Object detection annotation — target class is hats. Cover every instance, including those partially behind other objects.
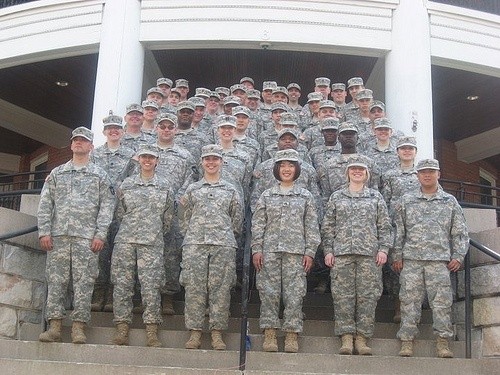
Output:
[72,77,440,171]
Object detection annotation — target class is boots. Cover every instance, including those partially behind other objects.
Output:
[103,288,114,313]
[132,303,144,314]
[355,336,373,354]
[262,327,278,351]
[90,289,104,312]
[161,293,176,315]
[184,330,203,349]
[394,299,401,322]
[38,319,62,344]
[340,334,354,355]
[70,320,86,344]
[146,323,163,348]
[211,328,226,350]
[107,323,129,345]
[435,337,453,358]
[284,332,299,353]
[399,341,412,357]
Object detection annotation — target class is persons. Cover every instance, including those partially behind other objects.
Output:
[86,77,450,324]
[249,149,321,354]
[320,163,392,355]
[39,125,117,344]
[109,144,173,350]
[391,160,472,358]
[177,140,248,350]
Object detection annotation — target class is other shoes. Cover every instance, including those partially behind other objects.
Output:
[314,280,327,293]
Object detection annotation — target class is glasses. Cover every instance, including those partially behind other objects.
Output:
[158,124,175,130]
